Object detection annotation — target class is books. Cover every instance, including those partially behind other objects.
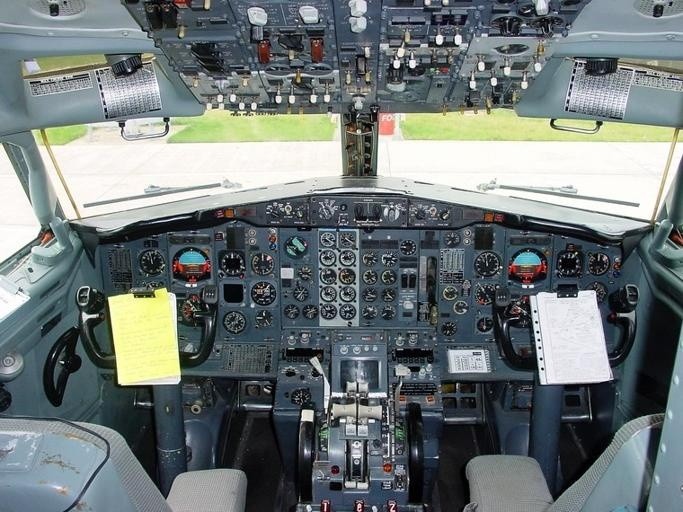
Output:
[527,289,615,387]
[105,285,182,389]
